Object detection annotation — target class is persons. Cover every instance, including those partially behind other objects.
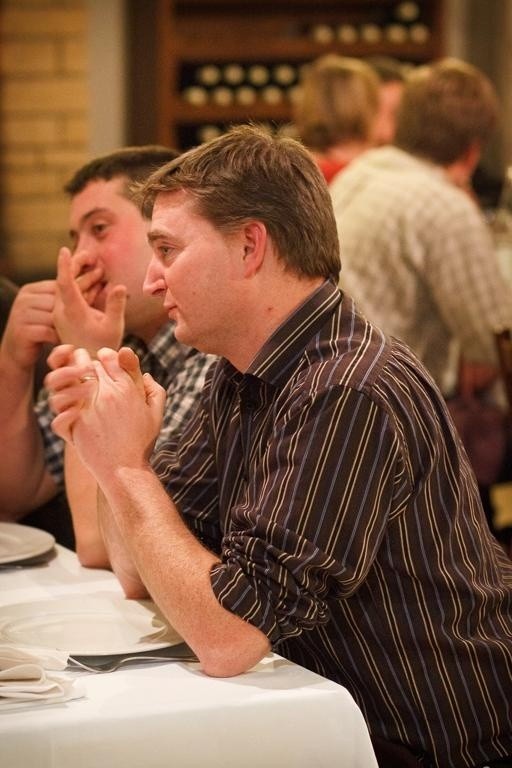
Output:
[48,127,511,766]
[2,146,223,569]
[292,56,512,485]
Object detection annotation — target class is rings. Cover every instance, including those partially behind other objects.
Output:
[79,373,96,384]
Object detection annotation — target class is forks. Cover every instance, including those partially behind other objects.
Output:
[68,655,202,673]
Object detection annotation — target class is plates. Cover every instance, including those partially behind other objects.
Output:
[0,594,186,656]
[0,519,54,564]
